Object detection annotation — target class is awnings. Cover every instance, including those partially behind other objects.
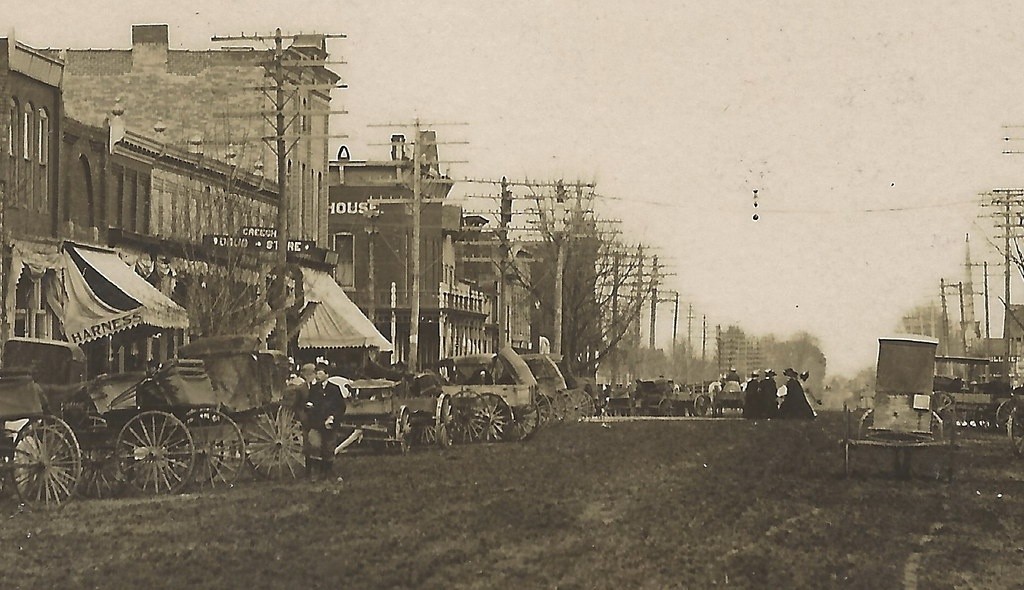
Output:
[58,242,190,349]
[300,268,394,354]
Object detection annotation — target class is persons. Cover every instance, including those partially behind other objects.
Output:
[726,367,741,383]
[776,367,817,419]
[594,373,681,417]
[308,365,345,487]
[757,369,779,418]
[293,363,317,482]
[741,369,761,418]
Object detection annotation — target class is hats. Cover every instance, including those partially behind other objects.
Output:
[764,368,777,377]
[783,368,798,378]
[731,367,736,372]
[751,369,760,377]
[315,362,328,374]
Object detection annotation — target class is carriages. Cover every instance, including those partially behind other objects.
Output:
[0,333,1024,514]
